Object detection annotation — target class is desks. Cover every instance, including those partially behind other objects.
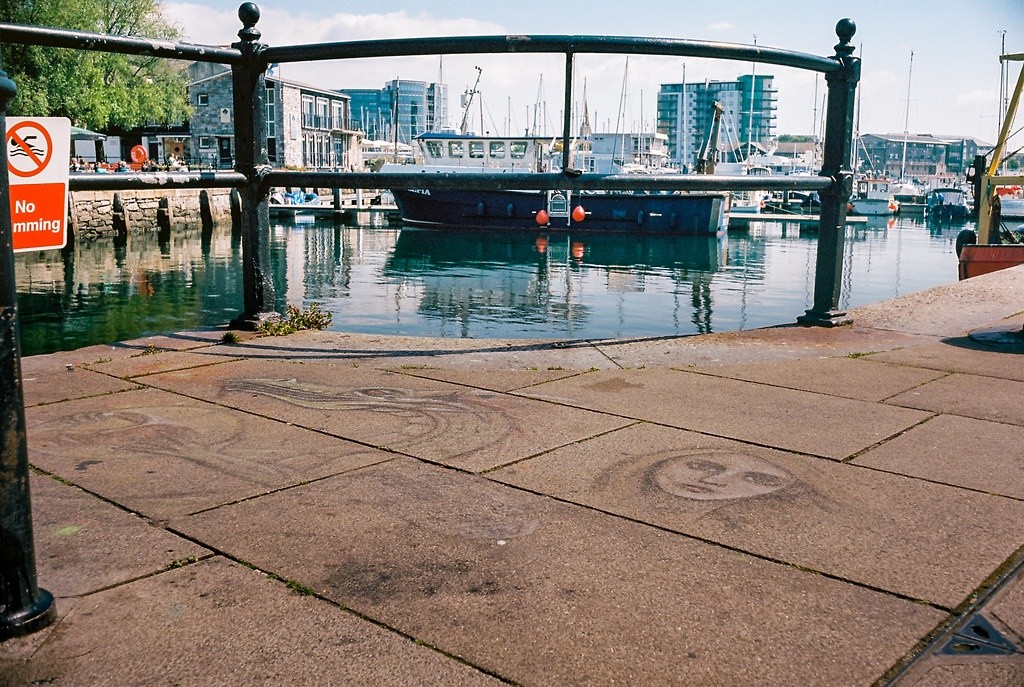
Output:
[157,165,213,172]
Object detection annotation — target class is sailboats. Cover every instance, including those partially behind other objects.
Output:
[268,29,1023,232]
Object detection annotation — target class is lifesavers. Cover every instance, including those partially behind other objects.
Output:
[130,144,147,164]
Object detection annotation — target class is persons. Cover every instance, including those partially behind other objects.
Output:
[168,153,181,173]
[115,161,129,172]
[69,157,80,171]
[95,162,109,174]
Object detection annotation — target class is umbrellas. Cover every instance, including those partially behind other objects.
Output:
[70,126,107,141]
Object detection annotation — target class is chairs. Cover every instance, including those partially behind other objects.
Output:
[74,156,218,173]
